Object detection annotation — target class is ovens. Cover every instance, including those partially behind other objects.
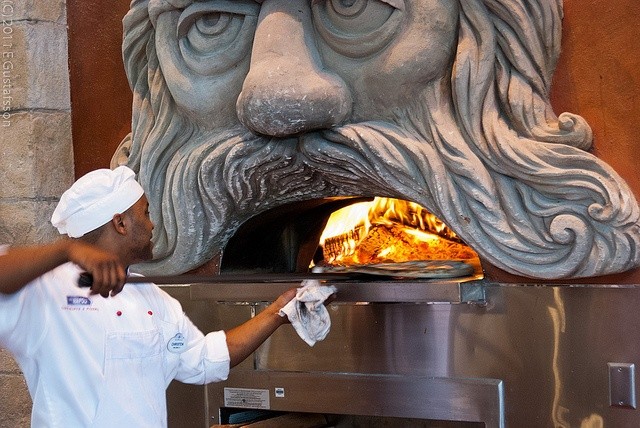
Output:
[118,273,638,428]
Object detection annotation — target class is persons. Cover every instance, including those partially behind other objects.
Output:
[0,165,337,428]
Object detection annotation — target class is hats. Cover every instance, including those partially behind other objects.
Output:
[51,166,145,239]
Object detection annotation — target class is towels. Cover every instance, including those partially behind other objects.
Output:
[279,272,339,352]
